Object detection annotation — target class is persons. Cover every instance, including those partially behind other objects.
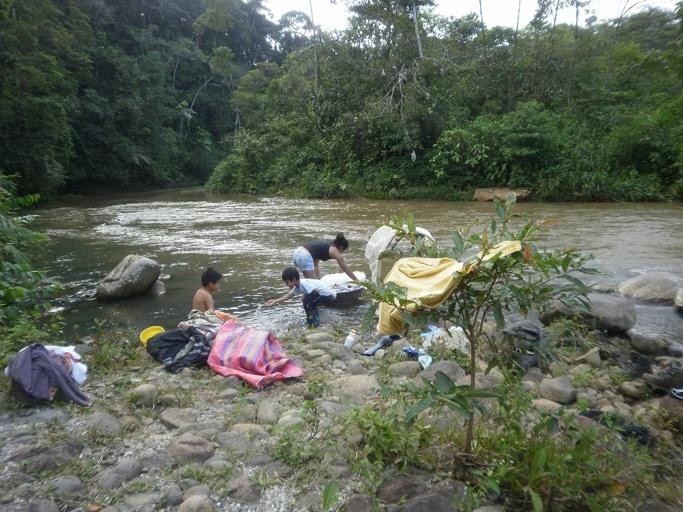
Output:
[191,267,224,314]
[264,266,336,330]
[289,232,365,285]
